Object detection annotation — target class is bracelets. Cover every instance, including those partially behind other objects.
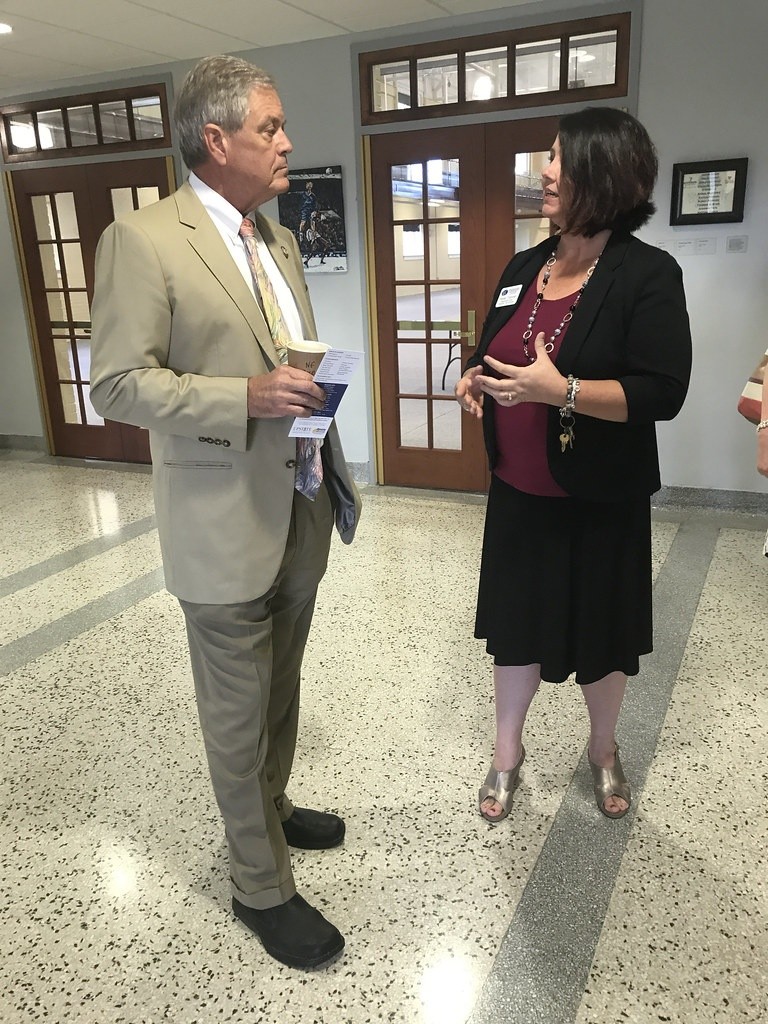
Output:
[756,420,768,432]
[558,372,580,417]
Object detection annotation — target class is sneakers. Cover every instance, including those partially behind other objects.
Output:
[231,892,346,971]
[283,806,346,849]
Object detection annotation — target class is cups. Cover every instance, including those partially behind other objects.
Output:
[285,341,335,376]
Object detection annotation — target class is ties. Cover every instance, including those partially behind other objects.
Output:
[240,217,323,502]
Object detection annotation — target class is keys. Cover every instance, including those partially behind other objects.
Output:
[557,416,575,453]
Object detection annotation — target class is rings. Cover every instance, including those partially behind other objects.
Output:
[507,393,512,401]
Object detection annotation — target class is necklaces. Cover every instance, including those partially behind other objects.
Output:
[522,246,604,363]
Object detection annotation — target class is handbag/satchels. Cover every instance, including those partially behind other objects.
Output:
[738,349,768,426]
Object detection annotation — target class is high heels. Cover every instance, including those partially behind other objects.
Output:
[479,743,525,822]
[588,741,631,818]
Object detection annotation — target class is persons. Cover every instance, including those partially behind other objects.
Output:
[87,52,362,969]
[454,107,694,823]
[298,182,330,269]
[756,363,768,480]
